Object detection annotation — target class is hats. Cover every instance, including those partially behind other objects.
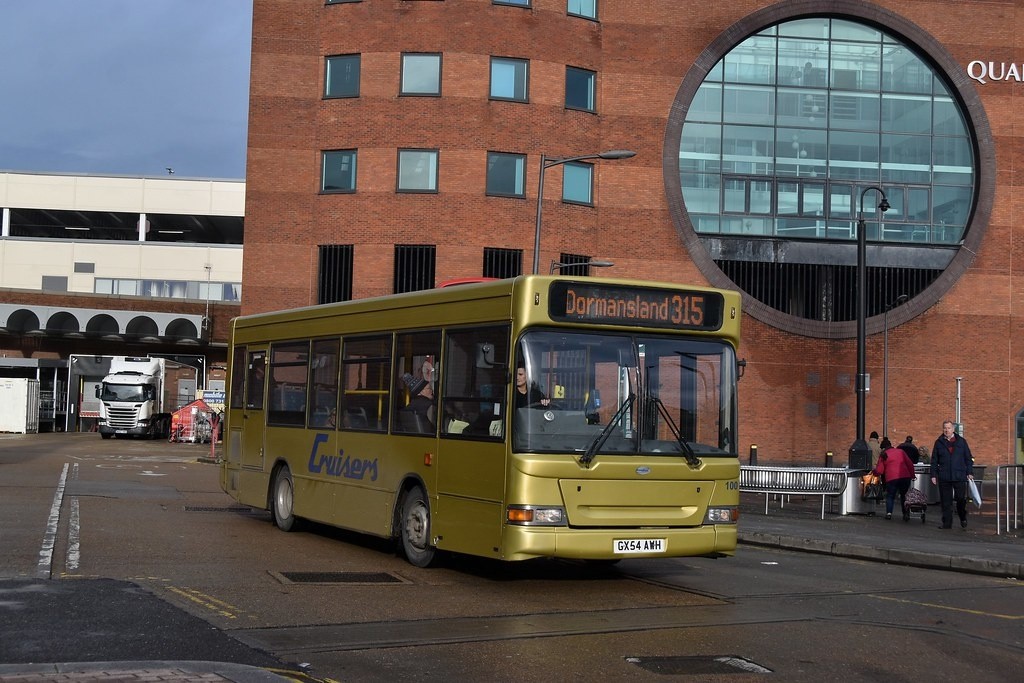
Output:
[870,431,878,439]
[402,372,429,395]
[880,437,891,448]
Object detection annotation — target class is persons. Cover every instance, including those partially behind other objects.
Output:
[868,431,930,469]
[403,373,438,433]
[875,437,917,521]
[325,408,336,428]
[240,358,276,406]
[515,368,550,410]
[930,420,974,529]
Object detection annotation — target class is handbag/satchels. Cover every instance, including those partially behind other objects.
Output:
[863,472,883,500]
[969,477,981,508]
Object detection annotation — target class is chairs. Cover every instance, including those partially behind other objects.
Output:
[234,379,471,434]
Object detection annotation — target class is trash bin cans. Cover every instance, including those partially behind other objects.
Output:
[838,469,876,516]
[910,462,940,503]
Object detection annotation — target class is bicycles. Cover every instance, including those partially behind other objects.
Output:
[168,426,184,443]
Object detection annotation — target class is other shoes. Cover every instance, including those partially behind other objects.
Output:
[939,524,951,530]
[886,512,892,519]
[960,517,967,528]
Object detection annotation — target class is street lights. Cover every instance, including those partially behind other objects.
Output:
[531,151,638,274]
[882,294,909,438]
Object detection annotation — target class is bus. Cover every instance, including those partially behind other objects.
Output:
[219,273,747,567]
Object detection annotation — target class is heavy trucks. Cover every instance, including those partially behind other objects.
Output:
[95,357,199,440]
